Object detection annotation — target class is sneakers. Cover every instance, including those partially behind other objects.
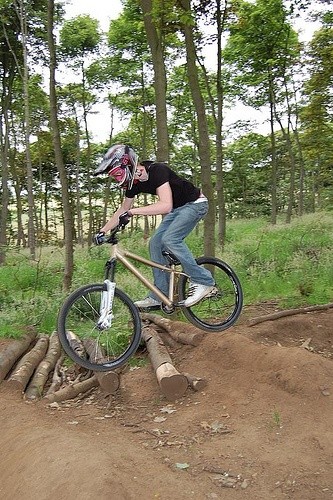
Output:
[133,297,166,310]
[185,283,214,307]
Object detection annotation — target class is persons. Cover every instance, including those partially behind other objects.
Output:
[92,144,215,307]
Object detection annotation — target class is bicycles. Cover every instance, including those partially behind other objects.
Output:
[57,211,244,372]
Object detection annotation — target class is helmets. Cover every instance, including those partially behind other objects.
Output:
[93,144,139,192]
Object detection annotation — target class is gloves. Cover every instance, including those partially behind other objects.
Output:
[94,232,106,245]
[117,212,130,226]
